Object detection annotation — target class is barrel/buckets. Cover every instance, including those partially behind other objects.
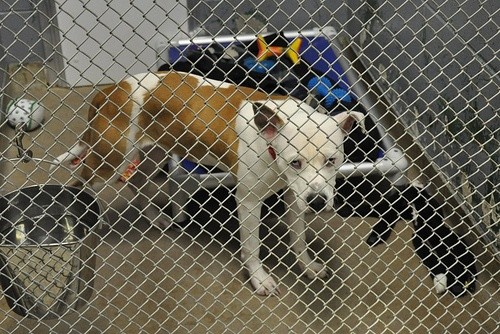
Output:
[1,184,102,320]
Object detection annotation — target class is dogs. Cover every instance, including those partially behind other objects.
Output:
[49,71,366,297]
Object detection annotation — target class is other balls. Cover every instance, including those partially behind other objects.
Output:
[6,97,44,132]
